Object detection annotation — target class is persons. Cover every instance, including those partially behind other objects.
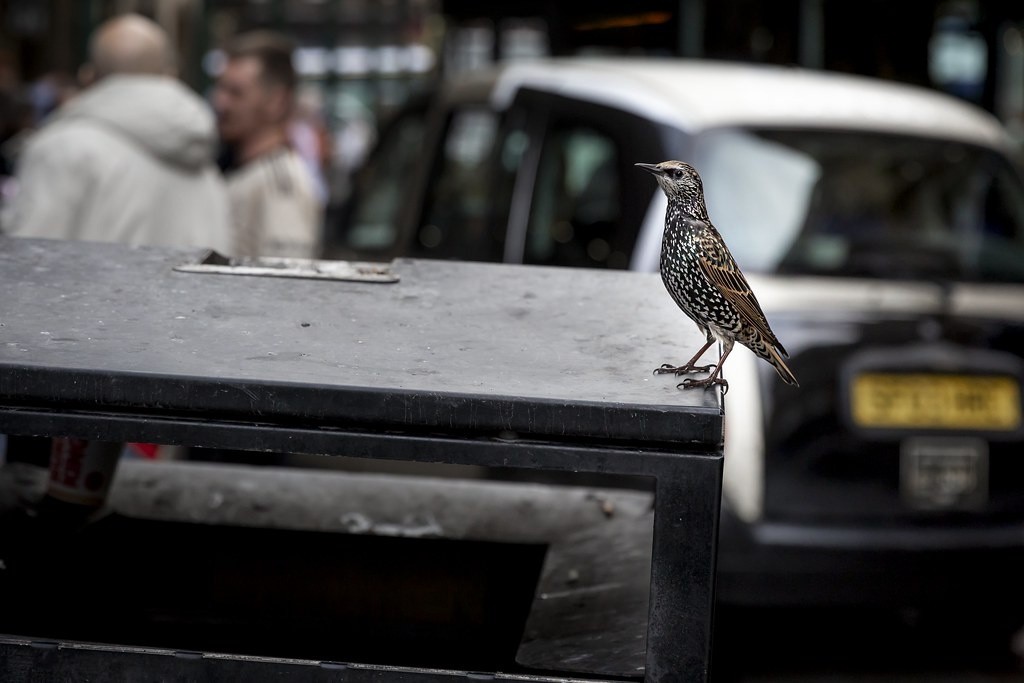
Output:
[1,11,232,253]
[213,29,329,257]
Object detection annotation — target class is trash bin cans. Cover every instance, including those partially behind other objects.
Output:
[0,237,724,683]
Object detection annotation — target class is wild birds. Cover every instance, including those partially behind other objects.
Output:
[634,159,800,394]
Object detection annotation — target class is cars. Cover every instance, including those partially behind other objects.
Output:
[316,54,1024,683]
